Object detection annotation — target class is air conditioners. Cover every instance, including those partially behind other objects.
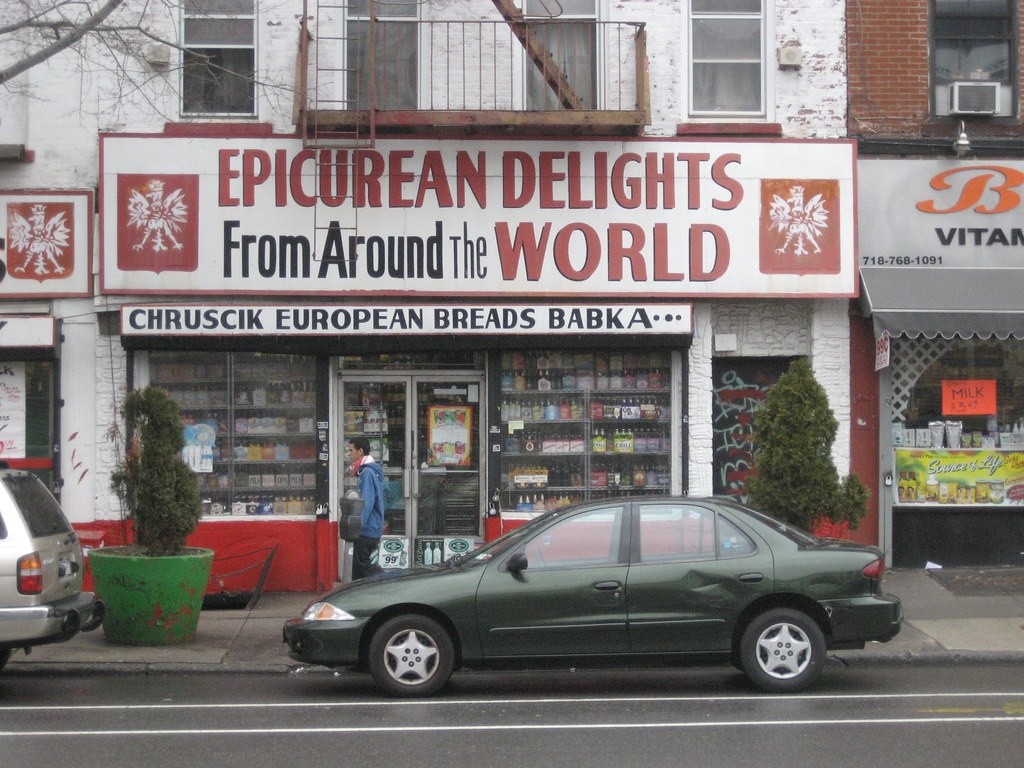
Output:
[948,80,1003,119]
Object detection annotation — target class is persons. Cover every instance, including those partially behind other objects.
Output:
[347,436,388,578]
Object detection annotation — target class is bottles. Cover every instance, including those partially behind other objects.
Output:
[150,344,677,521]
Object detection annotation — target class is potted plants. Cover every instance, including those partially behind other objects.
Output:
[85,385,214,647]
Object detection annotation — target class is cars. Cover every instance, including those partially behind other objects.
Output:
[0,461,103,672]
[279,495,904,699]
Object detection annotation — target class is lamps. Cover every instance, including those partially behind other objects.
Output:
[952,120,970,157]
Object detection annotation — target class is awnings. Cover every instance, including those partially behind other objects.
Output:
[857,265,1024,345]
